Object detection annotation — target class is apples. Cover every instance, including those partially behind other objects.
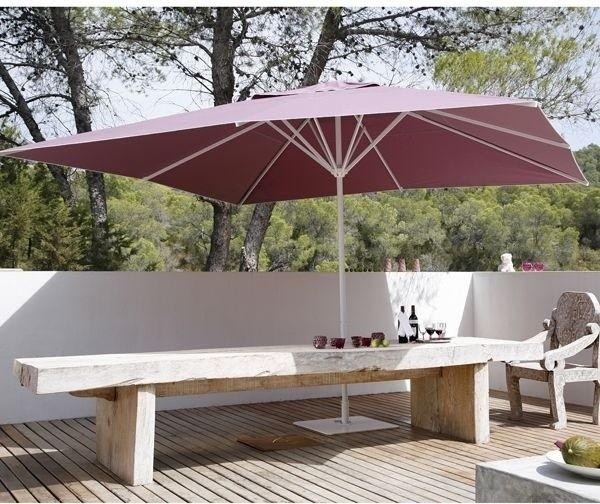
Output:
[382,339,389,347]
[371,339,379,347]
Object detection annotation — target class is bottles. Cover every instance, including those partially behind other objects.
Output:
[398,304,409,344]
[383,255,421,272]
[409,305,418,341]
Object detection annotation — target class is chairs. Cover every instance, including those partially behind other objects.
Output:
[501,289,598,432]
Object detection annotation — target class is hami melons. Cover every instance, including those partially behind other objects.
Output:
[562,435,600,468]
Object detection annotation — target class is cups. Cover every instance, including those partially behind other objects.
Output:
[522,262,545,271]
[312,332,387,350]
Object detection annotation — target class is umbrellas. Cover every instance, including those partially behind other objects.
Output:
[0,77,590,436]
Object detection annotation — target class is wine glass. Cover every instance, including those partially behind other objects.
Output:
[418,321,447,342]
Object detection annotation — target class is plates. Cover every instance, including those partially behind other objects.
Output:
[545,441,600,482]
